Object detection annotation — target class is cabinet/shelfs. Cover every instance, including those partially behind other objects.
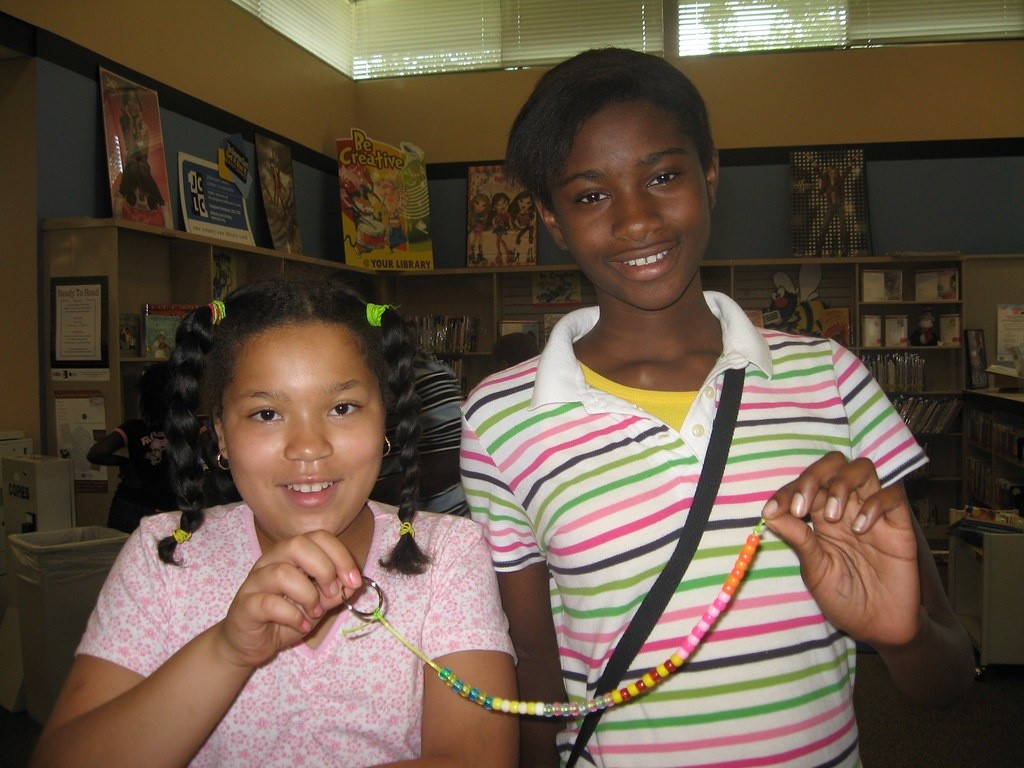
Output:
[40,211,1024,677]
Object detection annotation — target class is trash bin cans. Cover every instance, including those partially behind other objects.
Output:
[7,525,131,692]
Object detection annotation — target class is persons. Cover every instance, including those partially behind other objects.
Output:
[28,281,520,768]
[490,332,538,374]
[460,47,975,768]
[87,364,228,535]
[118,85,165,213]
[368,352,471,519]
[806,154,853,258]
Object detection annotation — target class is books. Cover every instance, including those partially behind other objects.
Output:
[968,407,1024,464]
[902,439,933,480]
[403,313,480,354]
[889,395,964,433]
[861,352,925,393]
[446,359,472,400]
[959,505,1024,534]
[968,457,1024,519]
[140,303,201,358]
[909,496,938,529]
